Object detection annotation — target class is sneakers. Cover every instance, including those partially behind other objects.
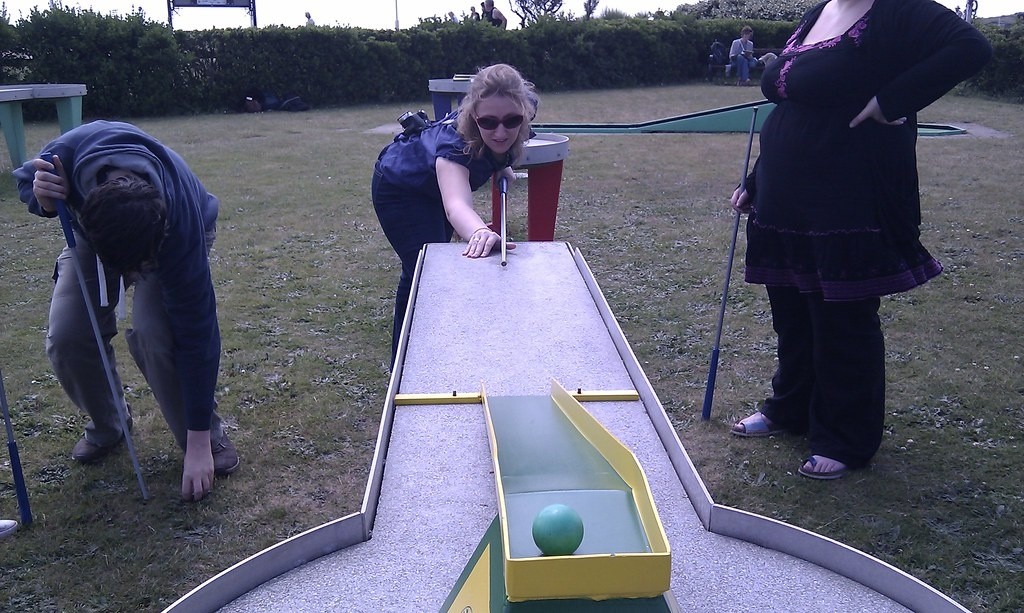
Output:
[0,520,18,538]
[211,431,239,474]
[71,402,133,463]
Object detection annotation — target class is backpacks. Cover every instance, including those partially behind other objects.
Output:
[711,42,728,64]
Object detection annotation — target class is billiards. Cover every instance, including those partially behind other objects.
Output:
[532,504,584,556]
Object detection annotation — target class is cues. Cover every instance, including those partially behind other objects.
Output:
[702,106,759,419]
[499,177,507,267]
[1,371,33,528]
[40,151,151,501]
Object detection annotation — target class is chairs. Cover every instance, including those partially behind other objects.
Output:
[707,40,765,81]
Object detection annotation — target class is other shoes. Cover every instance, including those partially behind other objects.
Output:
[744,79,751,85]
[737,81,742,86]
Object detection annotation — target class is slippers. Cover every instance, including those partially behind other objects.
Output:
[798,455,849,480]
[730,411,788,436]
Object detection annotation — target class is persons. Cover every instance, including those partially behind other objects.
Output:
[729,25,756,87]
[305,12,314,26]
[12,119,240,501]
[731,0,991,479]
[955,5,963,18]
[448,0,508,30]
[372,63,538,382]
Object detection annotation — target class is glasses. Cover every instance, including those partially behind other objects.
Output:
[475,112,523,130]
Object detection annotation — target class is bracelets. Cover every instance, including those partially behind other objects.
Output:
[471,227,492,235]
